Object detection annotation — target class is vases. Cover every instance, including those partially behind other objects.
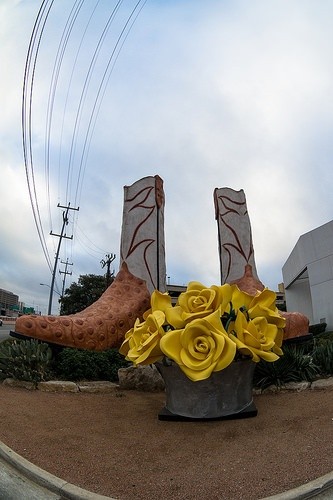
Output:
[152,361,259,422]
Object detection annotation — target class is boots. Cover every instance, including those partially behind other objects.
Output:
[214,186,313,344]
[10,174,168,352]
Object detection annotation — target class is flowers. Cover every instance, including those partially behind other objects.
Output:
[118,280,287,382]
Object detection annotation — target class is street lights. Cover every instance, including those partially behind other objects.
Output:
[39,282,64,315]
